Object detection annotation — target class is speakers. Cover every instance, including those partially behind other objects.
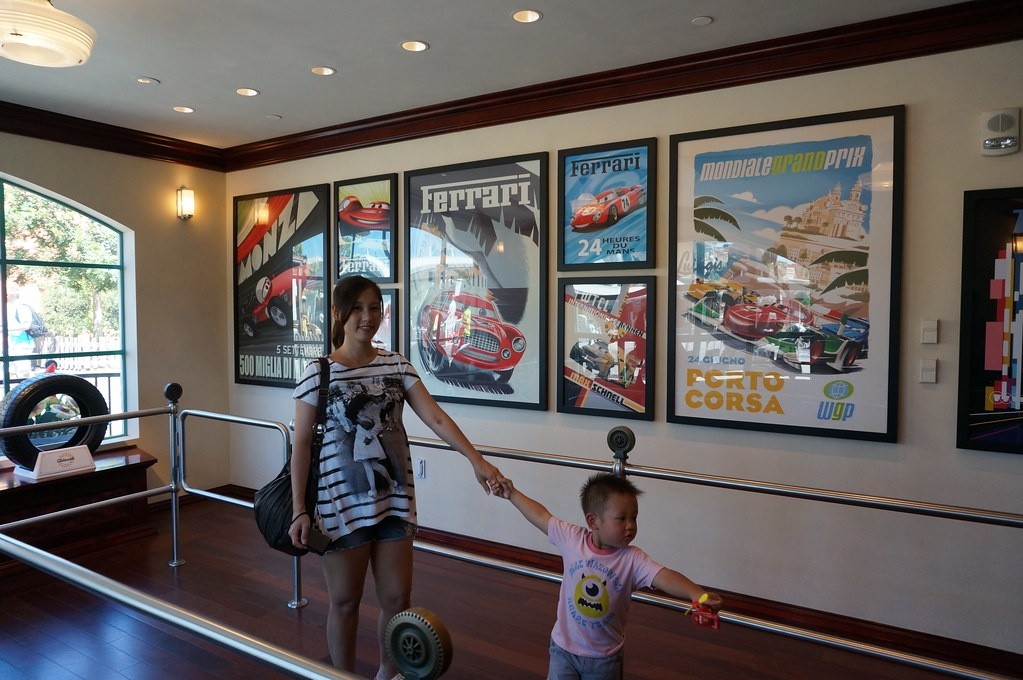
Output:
[979,108,1021,157]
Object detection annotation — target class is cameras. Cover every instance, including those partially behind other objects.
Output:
[298,529,332,556]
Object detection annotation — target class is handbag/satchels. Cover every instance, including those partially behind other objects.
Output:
[253,432,324,556]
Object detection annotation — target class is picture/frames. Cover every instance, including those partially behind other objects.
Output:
[370,288,399,353]
[403,151,549,412]
[666,103,906,445]
[233,182,331,389]
[956,186,1023,454]
[334,173,399,284]
[557,137,658,272]
[557,275,657,421]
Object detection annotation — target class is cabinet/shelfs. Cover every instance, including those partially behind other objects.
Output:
[0,444,159,578]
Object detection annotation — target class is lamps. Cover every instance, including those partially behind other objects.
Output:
[0,0,97,68]
[176,186,194,221]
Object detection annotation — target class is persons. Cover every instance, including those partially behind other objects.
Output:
[289,276,513,680]
[491,473,723,678]
